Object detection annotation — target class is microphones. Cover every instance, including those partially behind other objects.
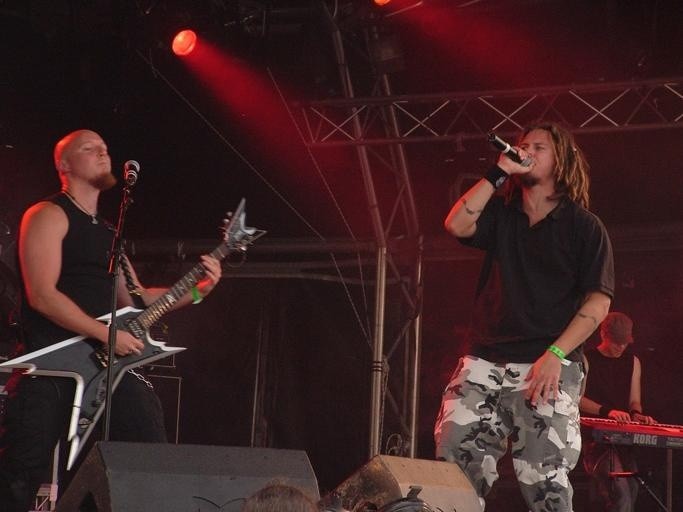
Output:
[489,133,532,167]
[124,160,140,184]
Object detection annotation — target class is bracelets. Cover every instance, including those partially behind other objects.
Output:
[548,345,566,361]
[484,163,510,189]
[631,410,641,415]
[599,407,610,418]
[191,287,203,305]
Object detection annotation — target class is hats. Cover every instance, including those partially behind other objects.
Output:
[601,312,634,344]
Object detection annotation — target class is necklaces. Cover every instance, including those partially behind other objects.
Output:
[62,189,99,224]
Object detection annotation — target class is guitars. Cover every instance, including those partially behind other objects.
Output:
[1,198,267,474]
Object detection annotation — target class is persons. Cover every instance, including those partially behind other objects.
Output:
[431,119,615,512]
[0,129,222,512]
[580,312,657,512]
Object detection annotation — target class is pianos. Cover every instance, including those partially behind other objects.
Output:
[578,416,683,451]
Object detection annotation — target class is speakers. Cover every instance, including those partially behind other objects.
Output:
[320,453,484,511]
[51,440,320,512]
